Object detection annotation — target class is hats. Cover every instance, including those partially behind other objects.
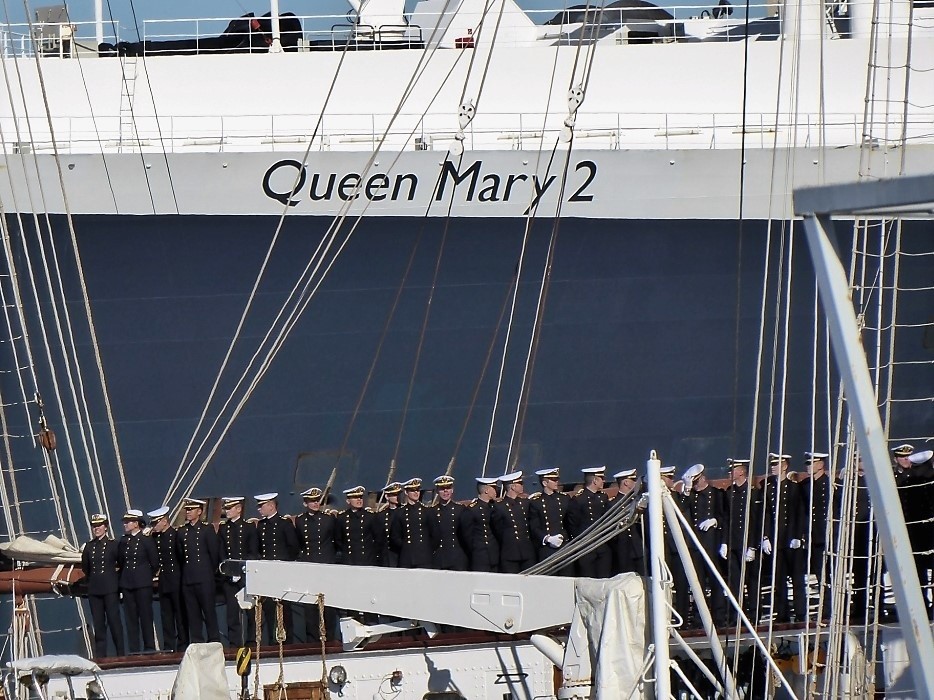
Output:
[475,466,676,487]
[300,488,323,501]
[91,514,109,526]
[343,475,455,497]
[122,510,144,521]
[254,493,278,507]
[804,444,934,468]
[147,506,170,524]
[727,458,750,473]
[769,453,792,466]
[222,497,245,510]
[183,498,207,509]
[682,464,704,485]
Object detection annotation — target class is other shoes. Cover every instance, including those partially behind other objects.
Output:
[774,617,790,623]
[794,617,805,621]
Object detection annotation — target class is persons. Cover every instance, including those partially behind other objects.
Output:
[81,445,934,659]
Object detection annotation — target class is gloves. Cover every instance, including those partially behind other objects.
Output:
[684,473,694,489]
[720,544,727,559]
[763,536,772,554]
[790,539,801,549]
[548,534,563,548]
[746,547,756,562]
[699,518,716,531]
